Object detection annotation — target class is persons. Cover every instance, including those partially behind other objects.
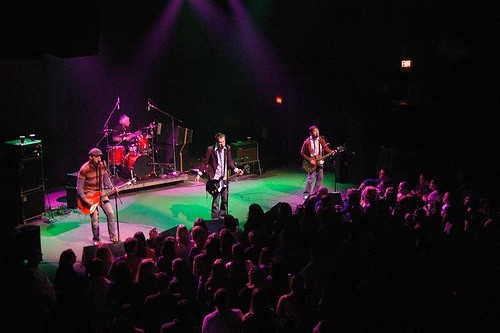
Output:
[25,167,500,333]
[77,147,120,245]
[112,115,135,146]
[300,125,335,200]
[195,133,243,220]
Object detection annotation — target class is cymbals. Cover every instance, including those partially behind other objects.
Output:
[96,129,117,134]
[142,125,160,130]
[116,132,132,138]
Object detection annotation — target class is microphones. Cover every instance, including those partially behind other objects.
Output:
[117,97,120,109]
[148,100,150,111]
[222,146,227,150]
[148,163,151,165]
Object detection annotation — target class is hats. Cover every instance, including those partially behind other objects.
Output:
[89,148,104,156]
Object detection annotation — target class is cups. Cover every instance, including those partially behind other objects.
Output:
[20,136,25,143]
[30,134,36,141]
[247,137,251,142]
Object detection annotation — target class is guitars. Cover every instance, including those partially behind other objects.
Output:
[77,177,137,215]
[302,146,344,173]
[205,164,252,198]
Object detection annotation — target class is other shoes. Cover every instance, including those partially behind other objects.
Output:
[93,239,100,246]
[111,237,120,241]
[302,195,309,199]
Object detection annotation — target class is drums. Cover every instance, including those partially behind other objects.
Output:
[107,145,126,165]
[134,134,149,152]
[123,153,155,179]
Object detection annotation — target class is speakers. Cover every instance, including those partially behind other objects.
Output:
[3,157,46,222]
[82,241,126,272]
[205,218,239,234]
[159,225,178,241]
[327,192,344,208]
[231,140,258,162]
